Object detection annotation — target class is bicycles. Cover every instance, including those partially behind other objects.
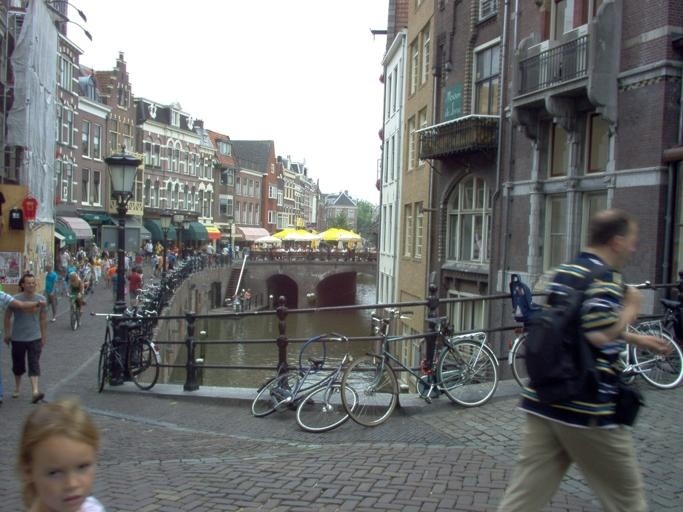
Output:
[65,293,83,332]
[85,308,164,395]
[122,252,208,324]
[248,332,360,436]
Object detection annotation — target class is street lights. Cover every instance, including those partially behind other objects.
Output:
[102,140,148,382]
[225,214,236,260]
[172,210,186,265]
[160,208,173,285]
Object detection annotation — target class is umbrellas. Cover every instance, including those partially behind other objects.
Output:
[272,228,360,242]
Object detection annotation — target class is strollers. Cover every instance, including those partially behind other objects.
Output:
[78,267,94,295]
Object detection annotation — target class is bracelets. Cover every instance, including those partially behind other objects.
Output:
[36,301,39,308]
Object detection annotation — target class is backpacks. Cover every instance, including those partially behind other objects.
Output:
[519,294,599,407]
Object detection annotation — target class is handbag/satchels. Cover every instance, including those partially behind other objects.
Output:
[610,378,644,427]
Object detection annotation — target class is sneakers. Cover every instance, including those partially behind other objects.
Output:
[51,318,57,322]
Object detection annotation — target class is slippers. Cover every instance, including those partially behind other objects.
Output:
[12,391,22,399]
[31,390,46,404]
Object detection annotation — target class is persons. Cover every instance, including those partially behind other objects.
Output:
[179,238,357,266]
[57,241,134,295]
[126,268,139,306]
[134,238,178,285]
[64,271,83,313]
[14,397,103,512]
[4,276,46,403]
[0,291,56,403]
[41,265,57,322]
[493,206,669,512]
